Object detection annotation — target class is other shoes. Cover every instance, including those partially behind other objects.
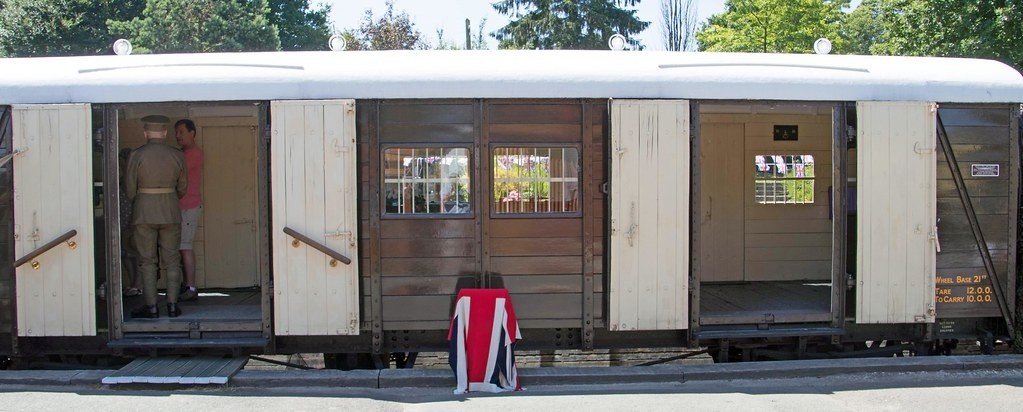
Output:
[97,285,107,300]
[180,286,199,301]
[124,287,143,296]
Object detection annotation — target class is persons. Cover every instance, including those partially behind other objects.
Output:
[119,115,204,318]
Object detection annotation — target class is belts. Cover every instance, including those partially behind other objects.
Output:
[139,187,178,194]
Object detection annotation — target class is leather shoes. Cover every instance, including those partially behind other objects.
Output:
[130,304,159,318]
[166,302,182,318]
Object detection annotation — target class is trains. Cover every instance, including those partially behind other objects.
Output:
[1,30,1023,365]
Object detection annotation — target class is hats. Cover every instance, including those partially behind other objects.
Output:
[141,115,171,132]
